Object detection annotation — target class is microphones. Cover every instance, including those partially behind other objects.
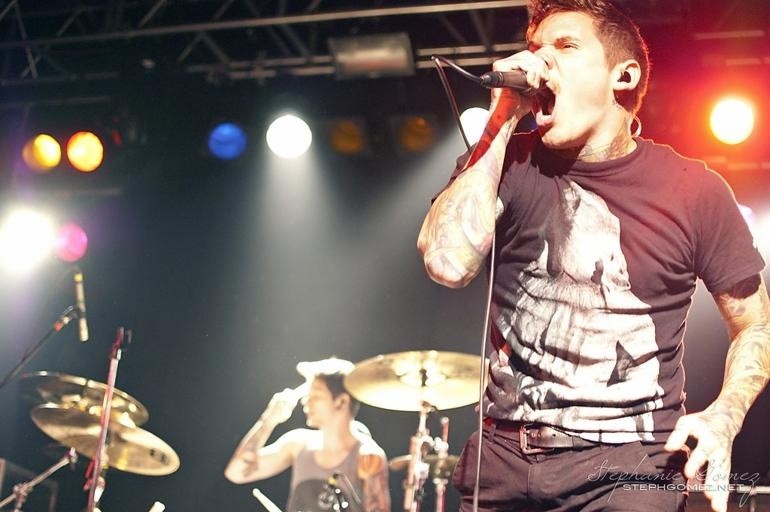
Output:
[477,69,527,91]
[71,262,90,344]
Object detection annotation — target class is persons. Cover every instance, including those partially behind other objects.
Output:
[413,0,770,511]
[224,355,393,512]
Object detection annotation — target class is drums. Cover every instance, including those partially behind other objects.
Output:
[0,458,59,511]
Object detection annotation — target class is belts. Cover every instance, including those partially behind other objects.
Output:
[480,415,596,457]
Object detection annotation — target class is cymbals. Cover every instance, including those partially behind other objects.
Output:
[343,350,491,412]
[390,453,461,477]
[13,371,149,426]
[30,402,181,476]
